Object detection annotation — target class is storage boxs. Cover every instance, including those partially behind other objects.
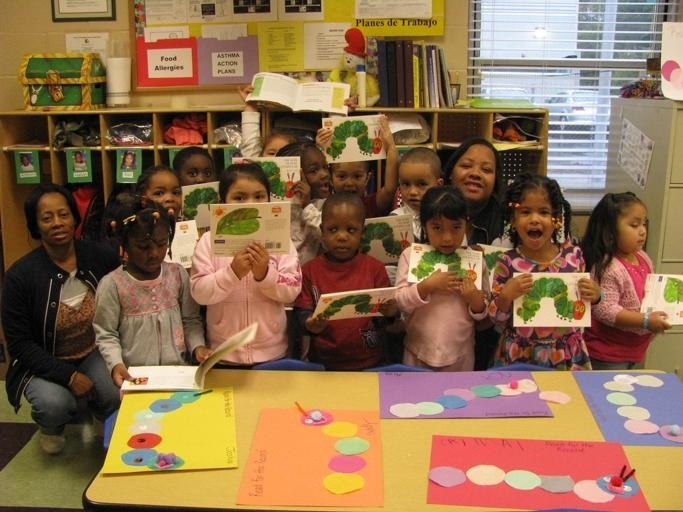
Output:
[19,54,107,111]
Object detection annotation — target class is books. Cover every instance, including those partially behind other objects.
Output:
[376,38,455,108]
[243,72,351,115]
[117,321,258,393]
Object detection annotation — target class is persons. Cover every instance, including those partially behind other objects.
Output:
[0,183,119,456]
[111,86,503,372]
[17,153,34,171]
[579,191,673,368]
[72,149,87,172]
[92,197,212,388]
[491,171,605,369]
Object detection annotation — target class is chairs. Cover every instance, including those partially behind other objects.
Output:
[363,364,433,372]
[487,363,554,371]
[251,359,325,371]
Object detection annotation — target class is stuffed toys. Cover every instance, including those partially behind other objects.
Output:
[327,26,381,108]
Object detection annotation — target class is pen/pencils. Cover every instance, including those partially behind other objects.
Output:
[294,401,307,416]
[194,389,213,396]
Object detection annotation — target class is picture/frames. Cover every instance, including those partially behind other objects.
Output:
[51,0,115,22]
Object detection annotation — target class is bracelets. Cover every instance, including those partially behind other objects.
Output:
[590,291,604,305]
[640,313,650,333]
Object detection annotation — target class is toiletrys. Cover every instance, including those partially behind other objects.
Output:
[355,63,367,108]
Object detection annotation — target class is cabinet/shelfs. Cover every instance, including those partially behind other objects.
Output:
[0,104,265,271]
[266,105,549,193]
[606,98,683,372]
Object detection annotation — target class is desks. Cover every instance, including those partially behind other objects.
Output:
[82,368,683,512]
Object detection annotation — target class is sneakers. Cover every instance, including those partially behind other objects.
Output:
[37,431,65,455]
